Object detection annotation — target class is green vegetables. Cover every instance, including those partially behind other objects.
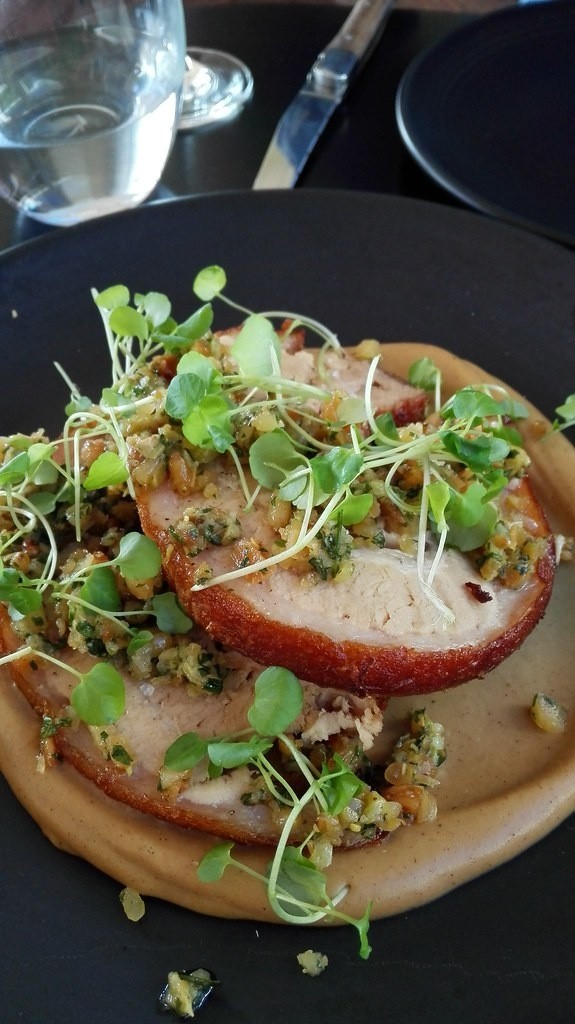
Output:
[0,264,575,959]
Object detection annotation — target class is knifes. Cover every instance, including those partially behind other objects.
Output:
[250,0,397,191]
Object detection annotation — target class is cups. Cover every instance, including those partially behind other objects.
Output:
[0,0,186,230]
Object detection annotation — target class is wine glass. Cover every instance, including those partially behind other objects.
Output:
[174,45,255,130]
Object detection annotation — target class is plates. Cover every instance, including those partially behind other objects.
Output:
[395,0,575,244]
[0,189,575,1024]
